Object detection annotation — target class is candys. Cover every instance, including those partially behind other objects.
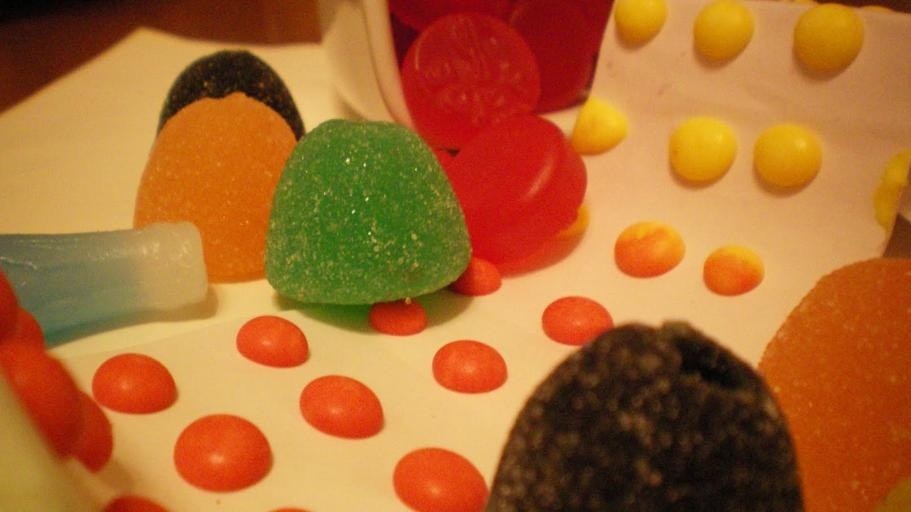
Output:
[0,0,911,512]
